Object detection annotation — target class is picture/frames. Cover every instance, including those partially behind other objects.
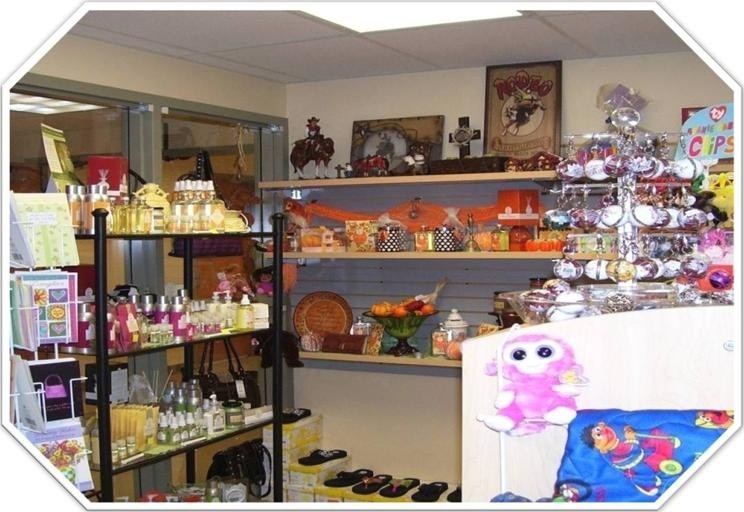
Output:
[482,59,563,163]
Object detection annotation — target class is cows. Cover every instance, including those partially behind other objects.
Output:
[289,136,335,180]
[501,97,546,139]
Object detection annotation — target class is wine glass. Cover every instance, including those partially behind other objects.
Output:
[362,310,441,356]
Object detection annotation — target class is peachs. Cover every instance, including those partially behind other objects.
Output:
[446,341,462,360]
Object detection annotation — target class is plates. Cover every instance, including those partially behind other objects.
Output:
[293,291,354,350]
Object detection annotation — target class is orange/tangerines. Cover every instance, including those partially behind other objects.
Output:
[395,307,408,318]
[420,305,434,314]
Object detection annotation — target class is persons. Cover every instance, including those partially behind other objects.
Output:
[304,117,320,151]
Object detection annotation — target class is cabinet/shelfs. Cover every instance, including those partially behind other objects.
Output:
[258,170,618,370]
[50,203,286,503]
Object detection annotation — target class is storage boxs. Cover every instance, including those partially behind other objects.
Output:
[262,416,320,502]
[345,490,409,503]
[316,484,355,503]
[287,457,351,503]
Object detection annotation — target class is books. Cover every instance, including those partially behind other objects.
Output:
[11,355,95,493]
[10,193,80,353]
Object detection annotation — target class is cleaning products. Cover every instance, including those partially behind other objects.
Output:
[210,290,220,302]
[158,378,225,444]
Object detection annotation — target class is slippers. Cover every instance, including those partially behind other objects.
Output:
[324,468,460,502]
[283,408,312,423]
[298,449,348,465]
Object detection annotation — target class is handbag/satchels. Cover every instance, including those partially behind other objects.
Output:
[194,336,261,408]
[206,438,274,498]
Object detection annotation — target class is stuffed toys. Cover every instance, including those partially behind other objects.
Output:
[216,264,274,304]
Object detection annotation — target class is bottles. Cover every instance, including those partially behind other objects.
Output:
[443,308,469,342]
[414,225,429,252]
[170,180,225,233]
[430,321,450,357]
[490,224,509,251]
[111,436,136,465]
[64,184,166,236]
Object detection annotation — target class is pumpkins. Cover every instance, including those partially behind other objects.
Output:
[371,301,393,317]
